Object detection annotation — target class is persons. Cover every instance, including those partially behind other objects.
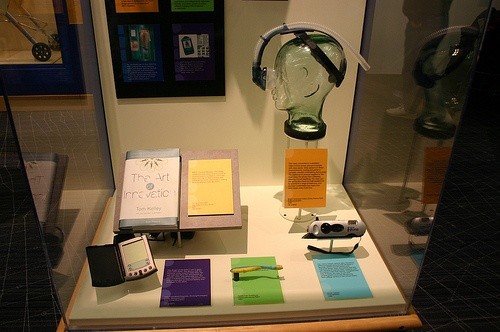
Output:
[413,26,481,138]
[270,33,346,141]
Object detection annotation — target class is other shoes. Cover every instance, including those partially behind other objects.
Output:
[385,104,418,121]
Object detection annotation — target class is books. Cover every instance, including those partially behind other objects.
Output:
[24,151,58,228]
[118,146,180,231]
